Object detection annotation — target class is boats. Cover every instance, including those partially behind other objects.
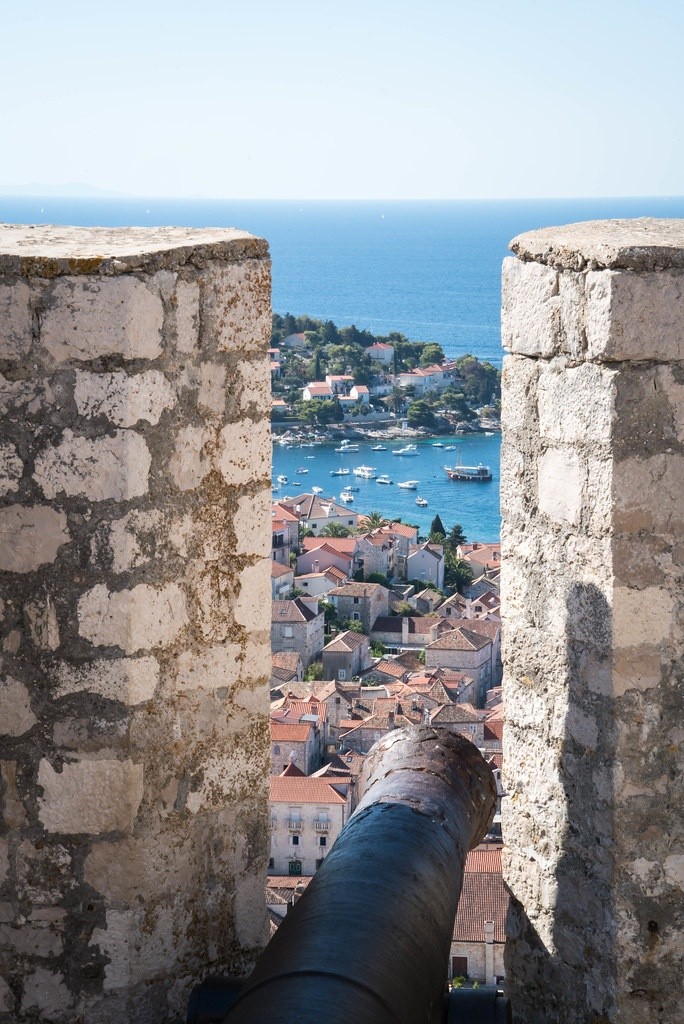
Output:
[440,448,493,482]
[272,432,456,508]
